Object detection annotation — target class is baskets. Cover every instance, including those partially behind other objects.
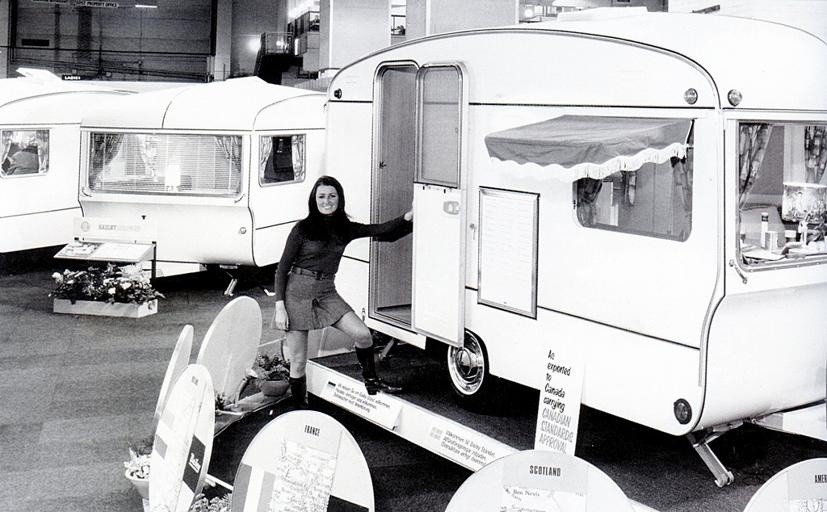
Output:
[258,367,289,396]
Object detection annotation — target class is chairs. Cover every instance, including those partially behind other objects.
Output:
[7,151,39,175]
[740,205,789,265]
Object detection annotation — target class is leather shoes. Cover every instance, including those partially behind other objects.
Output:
[291,267,331,279]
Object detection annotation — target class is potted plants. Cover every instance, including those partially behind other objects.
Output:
[257,351,291,396]
[123,448,151,498]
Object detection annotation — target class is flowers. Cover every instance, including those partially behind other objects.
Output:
[49,265,167,306]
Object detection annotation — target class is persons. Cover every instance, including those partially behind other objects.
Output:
[269,173,414,411]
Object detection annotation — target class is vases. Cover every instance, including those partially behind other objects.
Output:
[53,299,159,318]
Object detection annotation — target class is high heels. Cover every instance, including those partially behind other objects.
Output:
[365,377,404,395]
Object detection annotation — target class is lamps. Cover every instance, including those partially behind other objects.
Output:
[782,183,826,247]
